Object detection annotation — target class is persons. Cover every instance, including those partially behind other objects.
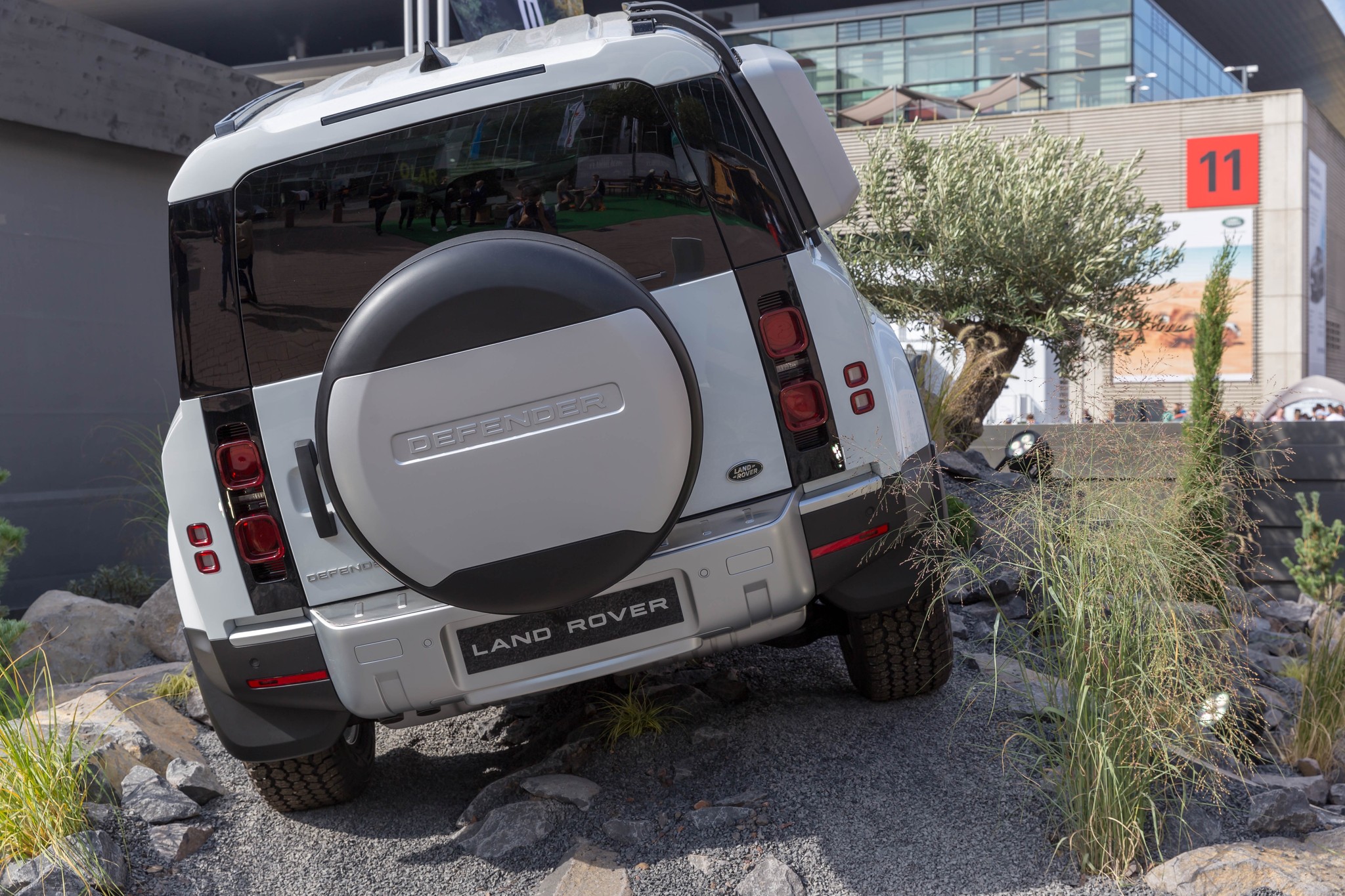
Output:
[290,186,346,224]
[1005,414,1040,424]
[207,198,258,308]
[1218,402,1345,421]
[170,219,195,388]
[1171,402,1187,420]
[644,168,672,200]
[370,172,607,234]
[1105,414,1114,423]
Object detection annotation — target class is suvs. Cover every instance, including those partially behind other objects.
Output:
[160,1,957,816]
